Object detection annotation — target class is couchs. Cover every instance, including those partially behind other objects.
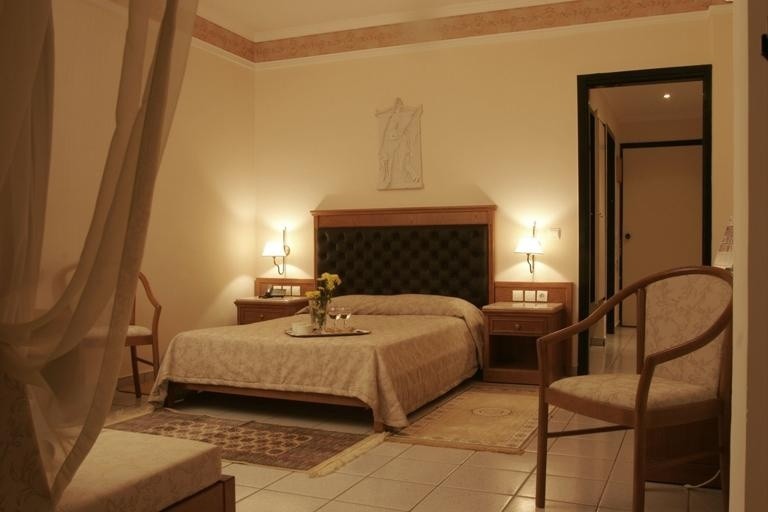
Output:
[0,340,236,512]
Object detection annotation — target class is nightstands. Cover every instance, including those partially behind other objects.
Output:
[481,302,565,386]
[234,296,309,327]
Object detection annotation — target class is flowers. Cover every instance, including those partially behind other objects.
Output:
[304,272,341,327]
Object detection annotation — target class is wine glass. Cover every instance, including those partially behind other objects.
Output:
[329,305,351,331]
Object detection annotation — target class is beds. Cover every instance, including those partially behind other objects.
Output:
[167,203,497,432]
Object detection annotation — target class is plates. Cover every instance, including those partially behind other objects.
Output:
[290,321,314,334]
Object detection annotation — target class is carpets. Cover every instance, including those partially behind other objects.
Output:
[102,406,387,478]
[385,379,560,456]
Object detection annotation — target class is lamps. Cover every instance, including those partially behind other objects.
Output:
[512,216,544,273]
[261,222,290,275]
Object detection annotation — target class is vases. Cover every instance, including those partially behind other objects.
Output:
[308,311,327,334]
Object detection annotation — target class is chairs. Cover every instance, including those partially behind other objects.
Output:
[54,260,165,405]
[534,265,733,511]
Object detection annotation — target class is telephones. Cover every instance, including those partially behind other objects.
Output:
[266,285,286,297]
